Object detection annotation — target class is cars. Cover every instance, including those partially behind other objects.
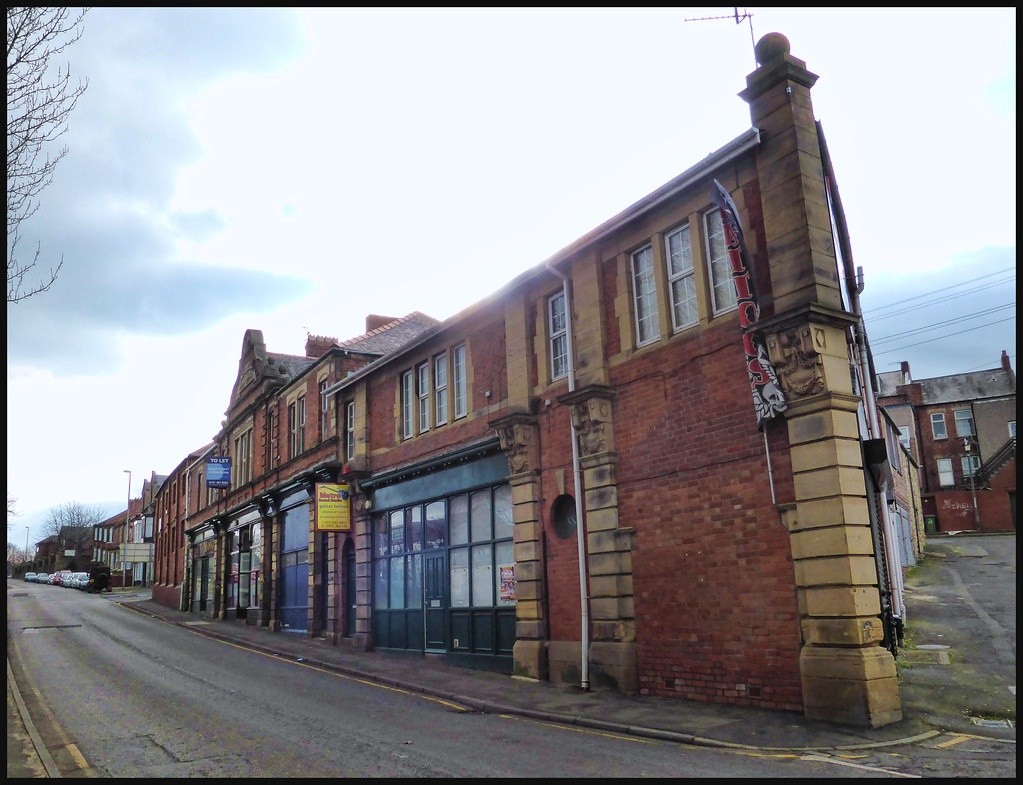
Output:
[35,573,49,583]
[60,573,71,586]
[64,575,72,588]
[71,572,87,588]
[76,573,89,591]
[24,572,36,582]
[48,574,54,585]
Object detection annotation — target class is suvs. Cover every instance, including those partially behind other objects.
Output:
[88,566,112,593]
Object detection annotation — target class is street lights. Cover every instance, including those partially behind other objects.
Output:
[123,470,132,588]
[964,438,981,531]
[25,526,29,562]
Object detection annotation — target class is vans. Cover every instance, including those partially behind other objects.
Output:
[53,570,72,585]
